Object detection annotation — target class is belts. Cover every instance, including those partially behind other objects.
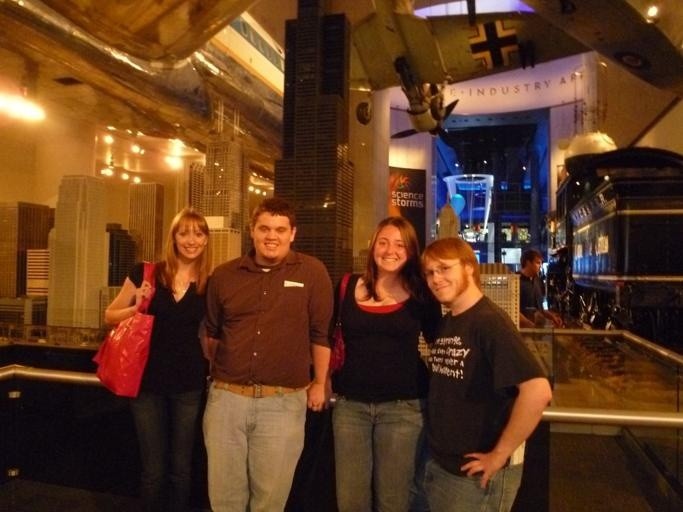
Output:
[214,381,303,397]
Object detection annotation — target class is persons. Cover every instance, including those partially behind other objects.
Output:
[516,250,562,328]
[199,198,335,512]
[325,217,443,511]
[103,208,214,512]
[546,245,577,304]
[416,236,552,512]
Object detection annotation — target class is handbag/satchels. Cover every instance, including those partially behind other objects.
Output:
[92,313,155,399]
[327,327,346,375]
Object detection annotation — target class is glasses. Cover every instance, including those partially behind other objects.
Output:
[423,263,462,278]
